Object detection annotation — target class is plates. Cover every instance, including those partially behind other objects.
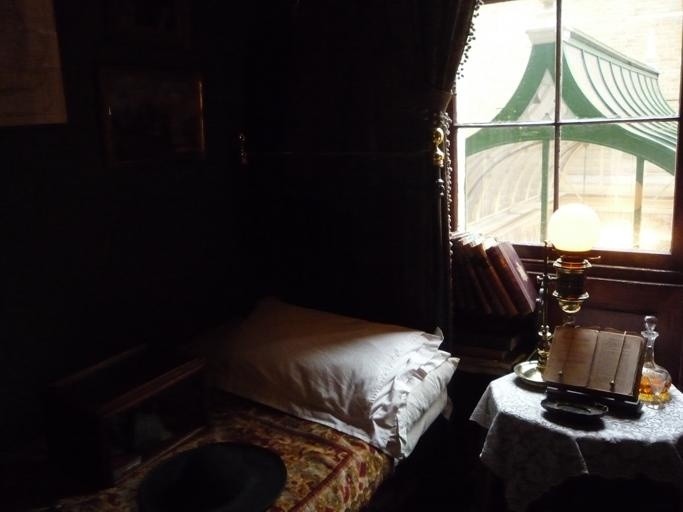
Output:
[540,397,609,421]
[513,360,554,388]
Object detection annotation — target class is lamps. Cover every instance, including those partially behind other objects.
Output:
[536,201,604,369]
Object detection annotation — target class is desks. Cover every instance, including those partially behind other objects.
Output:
[470,361,682,510]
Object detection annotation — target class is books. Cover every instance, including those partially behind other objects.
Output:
[451,229,536,315]
[540,324,647,401]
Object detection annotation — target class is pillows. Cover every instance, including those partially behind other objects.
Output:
[211,296,458,463]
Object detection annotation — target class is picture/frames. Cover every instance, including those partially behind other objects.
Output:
[94,63,204,169]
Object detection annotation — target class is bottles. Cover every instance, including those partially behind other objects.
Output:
[638,315,671,403]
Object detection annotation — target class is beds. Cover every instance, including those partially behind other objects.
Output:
[5,341,399,511]
